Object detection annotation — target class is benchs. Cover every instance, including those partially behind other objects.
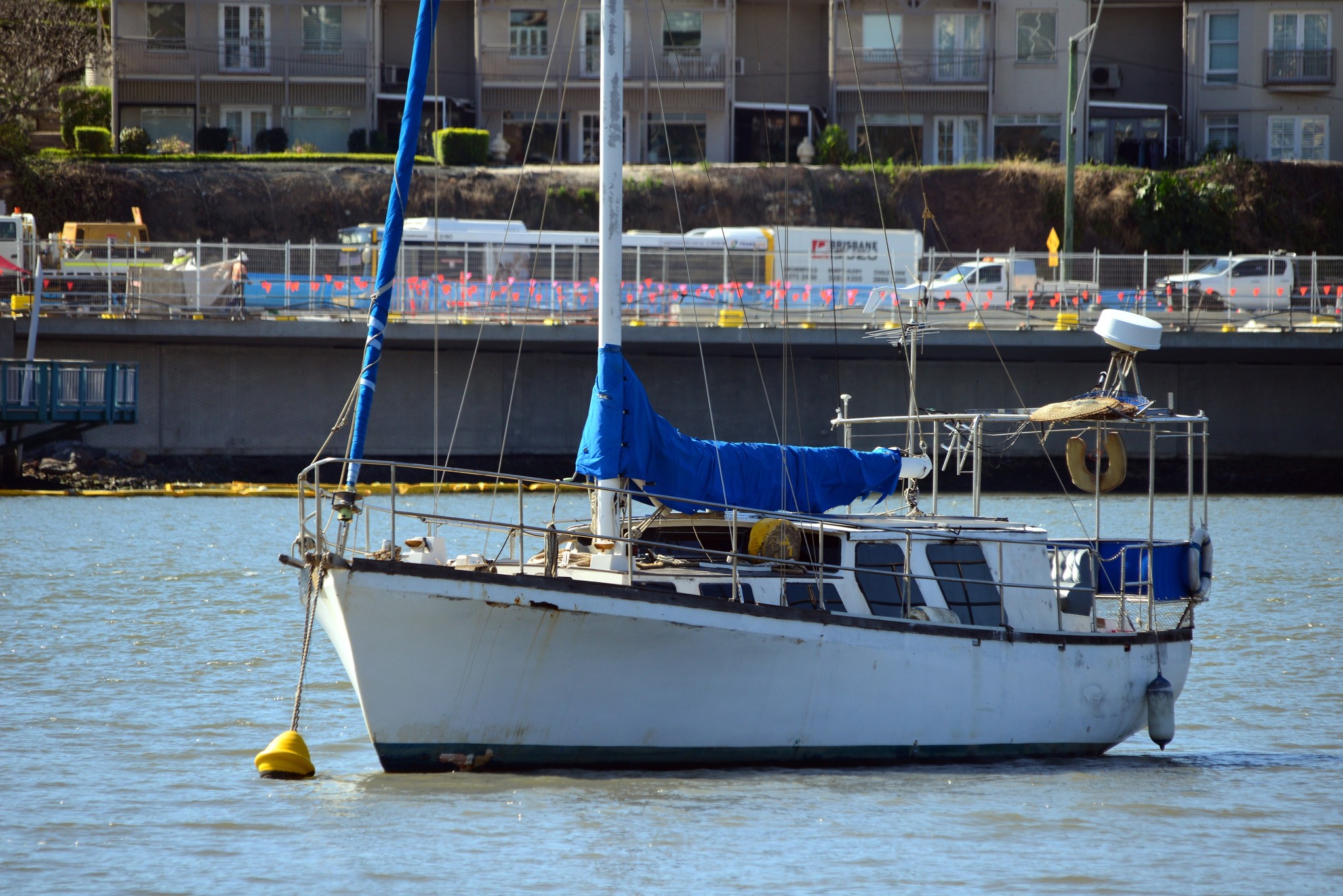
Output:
[1096,590,1193,604]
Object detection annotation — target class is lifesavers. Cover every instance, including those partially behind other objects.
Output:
[1065,431,1129,494]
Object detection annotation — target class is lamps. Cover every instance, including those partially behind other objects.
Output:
[404,539,424,549]
[593,541,615,550]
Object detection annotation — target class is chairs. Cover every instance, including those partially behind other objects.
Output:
[1049,548,1100,616]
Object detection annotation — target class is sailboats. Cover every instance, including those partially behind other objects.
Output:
[279,0,1215,783]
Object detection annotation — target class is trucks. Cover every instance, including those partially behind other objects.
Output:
[0,211,164,289]
[1152,254,1343,311]
[919,258,1102,311]
[686,226,924,294]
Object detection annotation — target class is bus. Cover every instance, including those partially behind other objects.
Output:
[331,215,778,310]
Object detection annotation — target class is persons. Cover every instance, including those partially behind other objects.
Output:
[224,251,252,315]
[172,247,194,266]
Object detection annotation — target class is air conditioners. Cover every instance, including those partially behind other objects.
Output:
[1090,61,1126,90]
